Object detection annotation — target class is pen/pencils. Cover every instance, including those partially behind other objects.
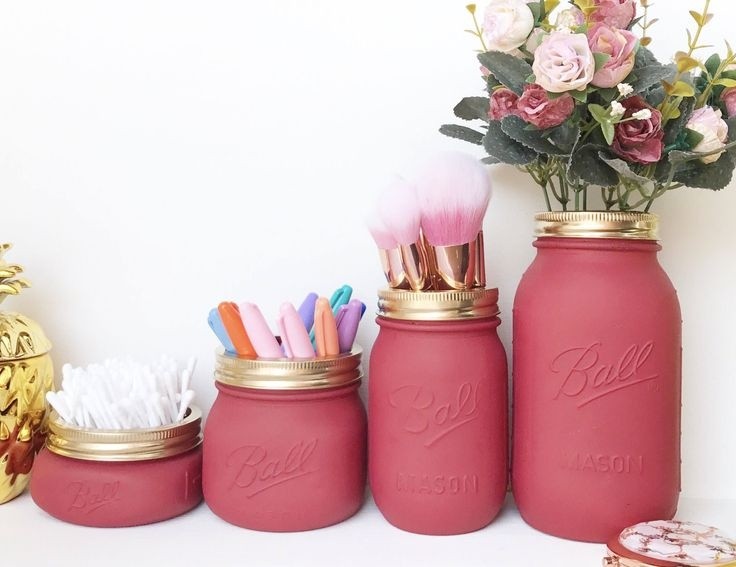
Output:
[205,284,365,360]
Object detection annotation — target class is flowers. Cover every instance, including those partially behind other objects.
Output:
[439,0,736,212]
